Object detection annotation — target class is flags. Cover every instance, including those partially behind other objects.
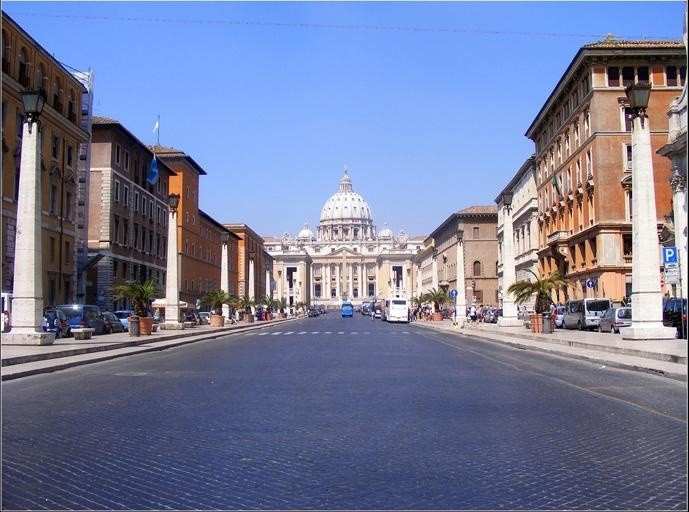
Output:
[147,154,159,185]
[153,119,159,132]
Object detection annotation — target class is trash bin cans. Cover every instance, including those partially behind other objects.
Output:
[128,315,140,336]
[542,312,551,334]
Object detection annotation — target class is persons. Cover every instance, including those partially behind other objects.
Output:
[621,295,631,307]
[408,306,422,323]
[466,303,496,323]
[371,302,376,320]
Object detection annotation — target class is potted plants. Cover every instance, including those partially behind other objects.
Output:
[104,278,157,336]
[199,289,233,328]
[409,287,451,321]
[237,294,310,322]
[504,266,577,334]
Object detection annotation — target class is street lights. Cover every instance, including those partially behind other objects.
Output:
[11,87,46,334]
[623,81,662,322]
[265,263,271,298]
[221,232,230,317]
[502,190,517,318]
[164,193,180,324]
[277,270,282,301]
[455,229,466,320]
[248,252,256,302]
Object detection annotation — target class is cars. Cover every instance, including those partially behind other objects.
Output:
[341,302,353,317]
[362,299,409,322]
[484,309,503,323]
[308,307,325,317]
[184,312,213,325]
[43,304,134,339]
[552,298,632,333]
[663,297,688,339]
